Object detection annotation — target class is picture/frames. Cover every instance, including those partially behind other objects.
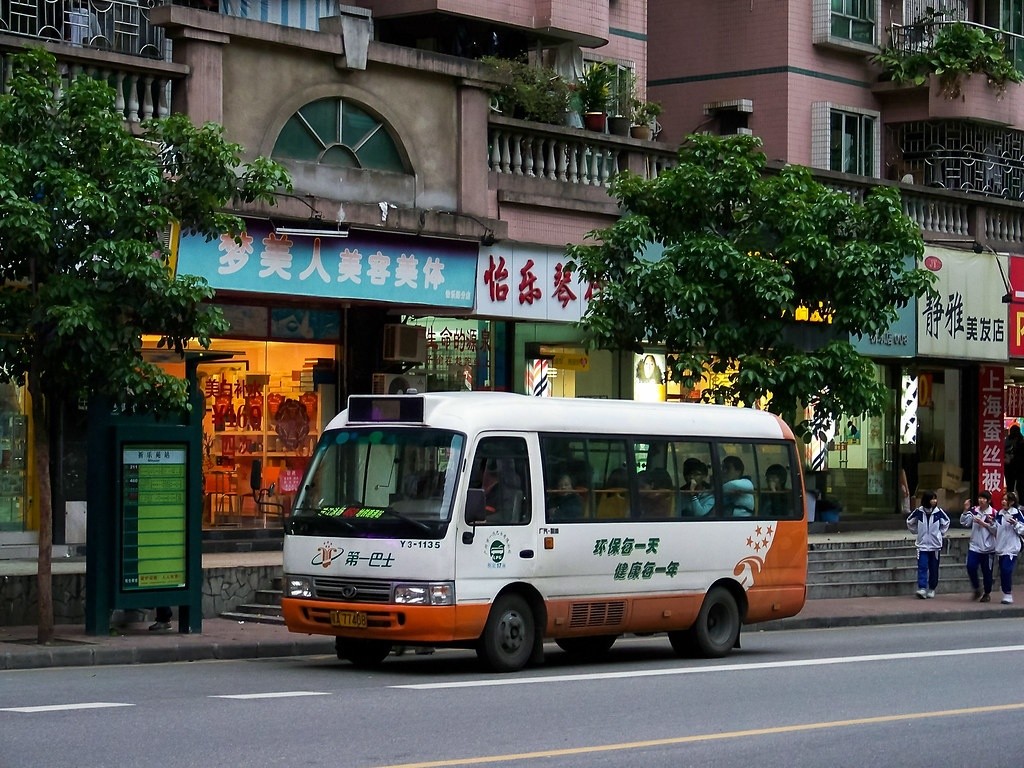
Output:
[198,360,249,412]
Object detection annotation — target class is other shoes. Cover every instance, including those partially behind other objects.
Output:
[980,594,992,603]
[974,590,985,601]
[1001,594,1013,603]
[1000,586,1005,596]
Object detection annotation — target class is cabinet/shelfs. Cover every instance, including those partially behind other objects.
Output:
[211,384,336,526]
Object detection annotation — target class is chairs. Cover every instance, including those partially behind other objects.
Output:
[204,466,284,529]
[493,473,523,524]
[574,486,759,518]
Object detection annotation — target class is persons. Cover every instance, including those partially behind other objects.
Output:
[549,474,583,521]
[987,492,1024,605]
[905,491,950,601]
[718,454,755,519]
[1002,425,1024,514]
[758,466,791,519]
[959,492,997,603]
[468,463,500,514]
[680,461,715,519]
[614,466,672,517]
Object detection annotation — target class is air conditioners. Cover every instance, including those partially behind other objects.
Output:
[382,323,427,364]
[372,373,427,394]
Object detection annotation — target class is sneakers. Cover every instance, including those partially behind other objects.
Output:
[916,589,926,599]
[927,590,936,598]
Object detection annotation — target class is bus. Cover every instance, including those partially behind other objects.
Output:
[249,388,808,674]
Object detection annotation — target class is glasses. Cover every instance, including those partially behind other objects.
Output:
[687,471,705,477]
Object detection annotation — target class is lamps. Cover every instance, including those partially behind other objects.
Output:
[269,220,349,238]
[925,238,984,254]
[989,248,1012,303]
[437,210,495,247]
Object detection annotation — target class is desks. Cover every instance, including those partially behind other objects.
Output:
[212,470,237,525]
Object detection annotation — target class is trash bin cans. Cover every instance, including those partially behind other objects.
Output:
[806,485,841,524]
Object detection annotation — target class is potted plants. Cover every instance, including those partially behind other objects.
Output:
[575,60,665,140]
[479,54,577,124]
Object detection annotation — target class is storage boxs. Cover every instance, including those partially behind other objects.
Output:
[911,462,971,513]
[265,358,334,393]
